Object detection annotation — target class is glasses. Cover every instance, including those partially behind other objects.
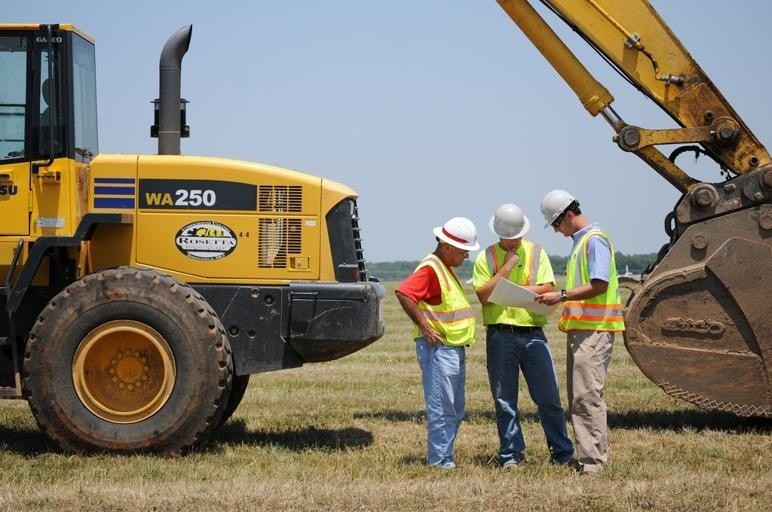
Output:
[551,215,565,227]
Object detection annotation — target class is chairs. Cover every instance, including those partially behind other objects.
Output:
[38,76,59,154]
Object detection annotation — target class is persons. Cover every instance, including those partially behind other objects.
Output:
[534,188,625,471]
[468,201,581,470]
[394,215,476,471]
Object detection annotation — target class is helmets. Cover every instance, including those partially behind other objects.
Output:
[541,190,574,228]
[433,218,479,252]
[489,204,529,240]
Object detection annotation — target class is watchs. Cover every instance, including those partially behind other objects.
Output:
[560,290,568,302]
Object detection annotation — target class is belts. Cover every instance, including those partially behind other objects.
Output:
[504,324,530,334]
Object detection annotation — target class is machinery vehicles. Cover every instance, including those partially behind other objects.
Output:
[0,23,389,457]
